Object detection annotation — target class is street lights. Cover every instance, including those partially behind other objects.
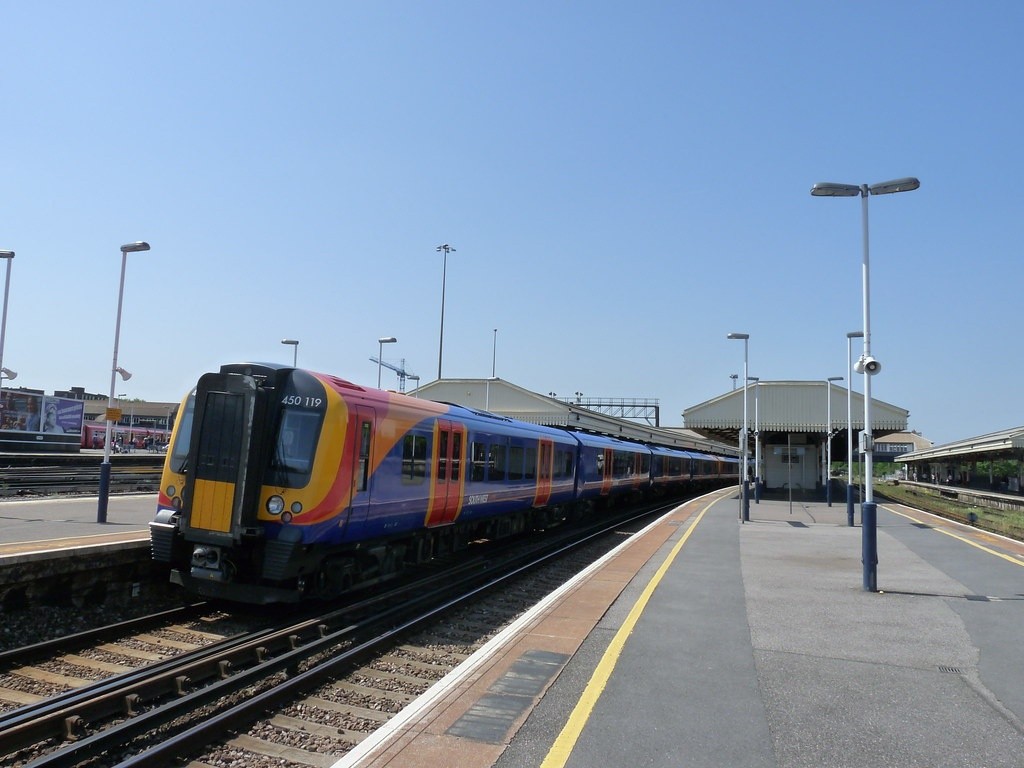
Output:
[726,333,750,525]
[376,336,397,388]
[746,376,760,505]
[0,249,17,386]
[827,375,844,508]
[809,177,922,588]
[435,243,456,381]
[127,401,134,453]
[96,242,152,523]
[847,330,865,527]
[485,376,500,410]
[280,338,299,369]
[406,376,419,399]
[113,394,127,454]
[162,406,170,447]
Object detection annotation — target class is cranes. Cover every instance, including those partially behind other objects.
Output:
[369,356,413,393]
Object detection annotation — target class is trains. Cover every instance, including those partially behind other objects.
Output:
[148,360,755,628]
[81,424,172,450]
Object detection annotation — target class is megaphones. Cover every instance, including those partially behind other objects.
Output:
[2,367,17,380]
[754,430,759,436]
[854,355,881,376]
[117,368,132,382]
